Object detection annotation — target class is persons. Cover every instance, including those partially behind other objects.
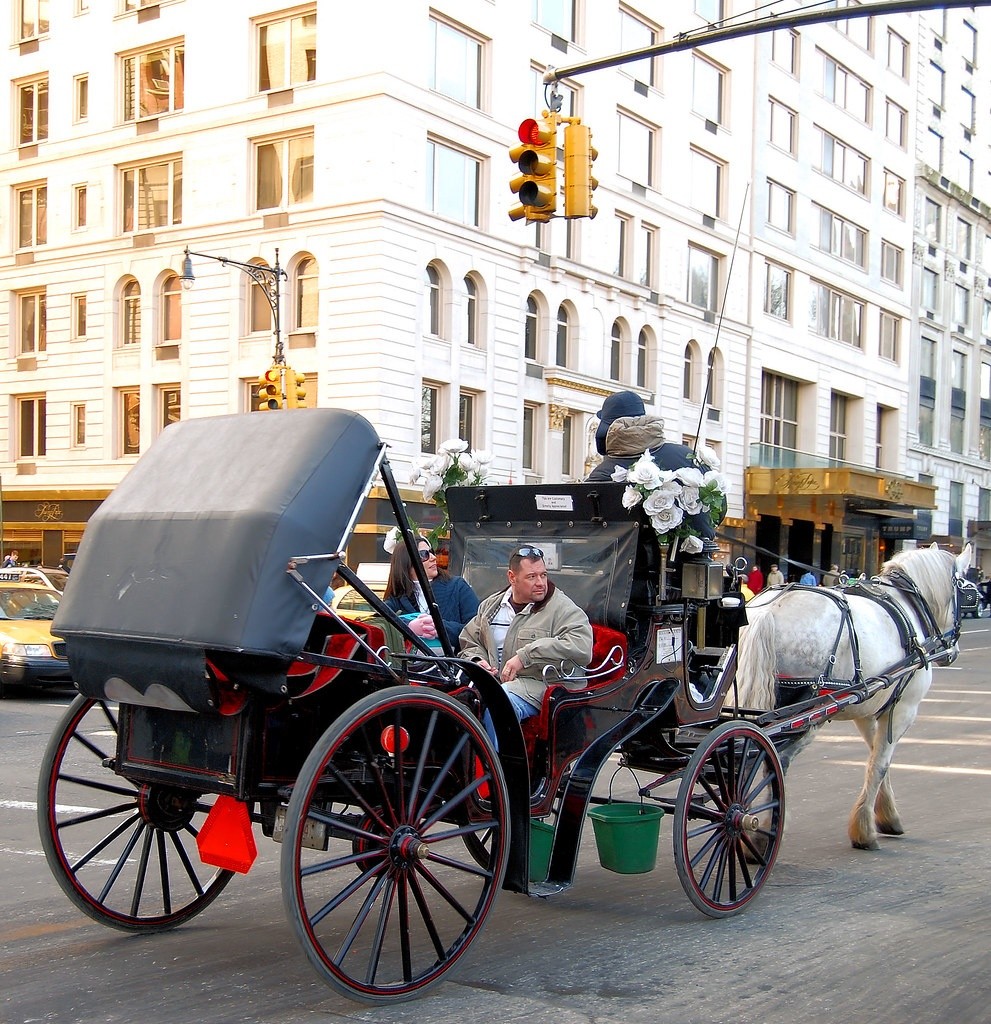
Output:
[318,571,345,612]
[738,566,763,601]
[766,565,784,588]
[58,558,71,574]
[457,545,592,753]
[383,536,480,684]
[585,390,728,588]
[823,564,861,587]
[1,550,19,569]
[800,571,816,586]
[976,565,991,618]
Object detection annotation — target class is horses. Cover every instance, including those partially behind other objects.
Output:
[725,540,972,847]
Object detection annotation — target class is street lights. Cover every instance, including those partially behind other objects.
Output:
[179,248,283,365]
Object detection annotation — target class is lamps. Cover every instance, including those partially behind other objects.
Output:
[809,496,818,514]
[828,499,837,518]
[777,494,783,509]
[879,528,886,550]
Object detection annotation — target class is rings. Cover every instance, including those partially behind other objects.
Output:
[505,669,511,673]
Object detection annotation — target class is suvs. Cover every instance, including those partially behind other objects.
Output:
[0,565,68,616]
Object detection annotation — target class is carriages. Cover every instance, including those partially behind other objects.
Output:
[37,407,973,1008]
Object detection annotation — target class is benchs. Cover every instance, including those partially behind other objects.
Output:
[210,612,387,706]
[402,611,629,755]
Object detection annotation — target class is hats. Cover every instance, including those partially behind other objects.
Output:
[595,391,645,455]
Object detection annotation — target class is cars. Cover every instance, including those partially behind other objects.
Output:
[320,582,388,620]
[0,581,77,695]
[958,568,985,618]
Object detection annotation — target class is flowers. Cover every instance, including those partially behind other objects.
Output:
[611,441,729,552]
[381,436,503,554]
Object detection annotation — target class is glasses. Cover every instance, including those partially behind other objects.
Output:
[508,548,544,562]
[419,548,436,562]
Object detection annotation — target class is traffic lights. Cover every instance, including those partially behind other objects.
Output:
[509,110,556,224]
[283,368,307,409]
[257,369,284,411]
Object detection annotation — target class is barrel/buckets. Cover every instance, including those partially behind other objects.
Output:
[528,818,555,883]
[587,762,665,874]
